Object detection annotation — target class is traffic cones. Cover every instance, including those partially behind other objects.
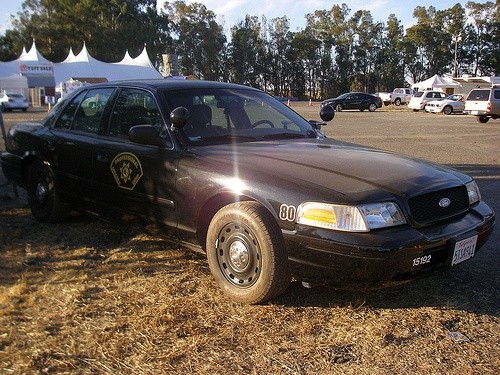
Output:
[308,98,313,107]
[287,99,291,107]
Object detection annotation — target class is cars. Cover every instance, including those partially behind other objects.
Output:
[0,78,496,306]
[320,92,383,112]
[425,94,467,115]
[407,90,450,112]
[0,92,29,112]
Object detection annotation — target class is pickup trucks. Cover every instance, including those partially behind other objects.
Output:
[379,87,411,106]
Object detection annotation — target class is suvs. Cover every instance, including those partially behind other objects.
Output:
[462,88,500,123]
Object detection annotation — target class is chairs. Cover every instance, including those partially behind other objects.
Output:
[65,106,103,132]
[120,105,149,135]
[189,104,224,137]
